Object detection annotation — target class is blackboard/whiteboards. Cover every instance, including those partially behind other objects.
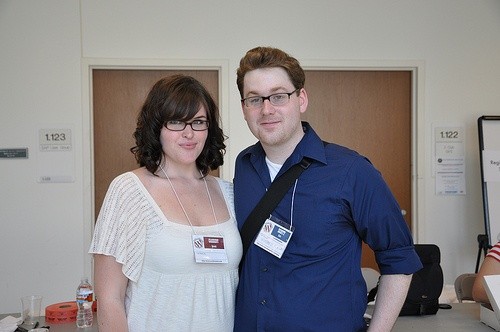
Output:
[482,120,500,246]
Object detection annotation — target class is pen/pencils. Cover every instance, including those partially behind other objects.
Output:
[33,321,39,328]
[21,318,27,325]
[40,326,50,329]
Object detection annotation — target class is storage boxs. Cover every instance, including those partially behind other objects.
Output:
[480,274,500,332]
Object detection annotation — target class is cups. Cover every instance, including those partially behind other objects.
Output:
[21,296,41,325]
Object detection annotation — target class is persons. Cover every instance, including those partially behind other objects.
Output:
[471,241,500,304]
[231,47,422,332]
[88,74,244,332]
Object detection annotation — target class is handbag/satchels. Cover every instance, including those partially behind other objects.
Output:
[367,245,444,318]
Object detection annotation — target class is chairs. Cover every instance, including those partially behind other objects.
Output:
[455,274,477,303]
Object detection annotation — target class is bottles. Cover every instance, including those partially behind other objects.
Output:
[75,277,93,329]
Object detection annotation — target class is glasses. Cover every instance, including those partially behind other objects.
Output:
[241,87,299,108]
[162,119,210,132]
